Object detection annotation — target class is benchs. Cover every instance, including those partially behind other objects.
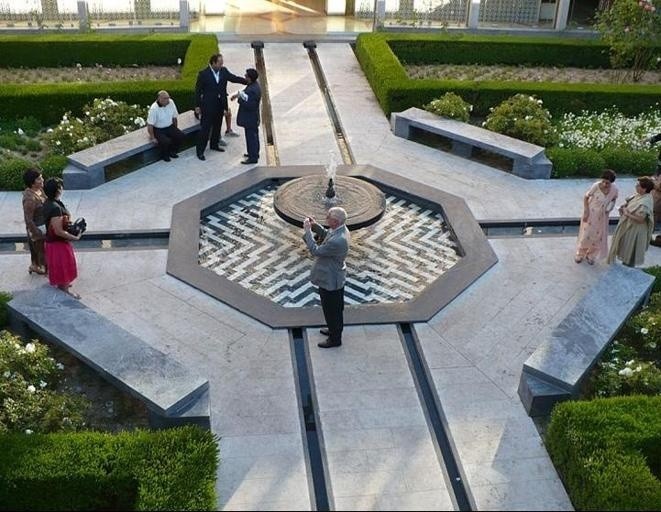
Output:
[389,105,555,181]
[4,279,215,431]
[516,260,660,415]
[60,105,206,190]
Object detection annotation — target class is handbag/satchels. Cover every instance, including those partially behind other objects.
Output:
[72,218,86,236]
[28,224,47,242]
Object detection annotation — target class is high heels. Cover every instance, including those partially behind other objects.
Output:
[29,266,46,274]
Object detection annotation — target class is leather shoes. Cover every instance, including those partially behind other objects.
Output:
[211,148,225,152]
[163,156,170,161]
[241,160,257,164]
[170,154,178,158]
[318,340,338,347]
[320,329,329,335]
[197,154,205,160]
[244,154,259,158]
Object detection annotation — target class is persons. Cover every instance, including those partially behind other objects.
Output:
[301,206,350,348]
[43,177,82,299]
[574,169,619,265]
[22,168,48,275]
[650,164,661,247]
[146,90,184,161]
[230,69,261,164]
[217,110,240,146]
[193,54,248,161]
[640,133,661,164]
[606,175,655,268]
